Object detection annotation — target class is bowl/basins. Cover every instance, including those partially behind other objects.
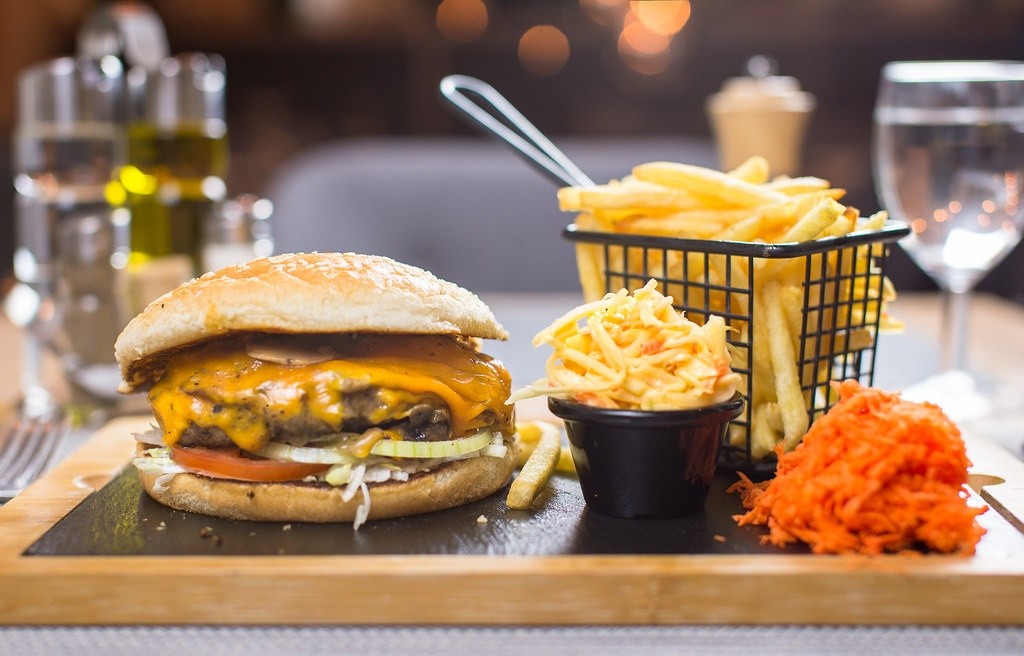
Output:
[549,392,747,520]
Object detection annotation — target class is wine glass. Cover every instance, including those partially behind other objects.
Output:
[871,59,1023,440]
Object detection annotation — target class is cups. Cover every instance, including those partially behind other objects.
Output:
[12,52,276,421]
[705,73,818,179]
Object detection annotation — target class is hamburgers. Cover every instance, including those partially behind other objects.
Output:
[112,250,517,530]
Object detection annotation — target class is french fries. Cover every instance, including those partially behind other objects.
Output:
[507,158,897,510]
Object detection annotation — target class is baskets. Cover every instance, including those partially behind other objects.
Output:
[563,216,914,479]
